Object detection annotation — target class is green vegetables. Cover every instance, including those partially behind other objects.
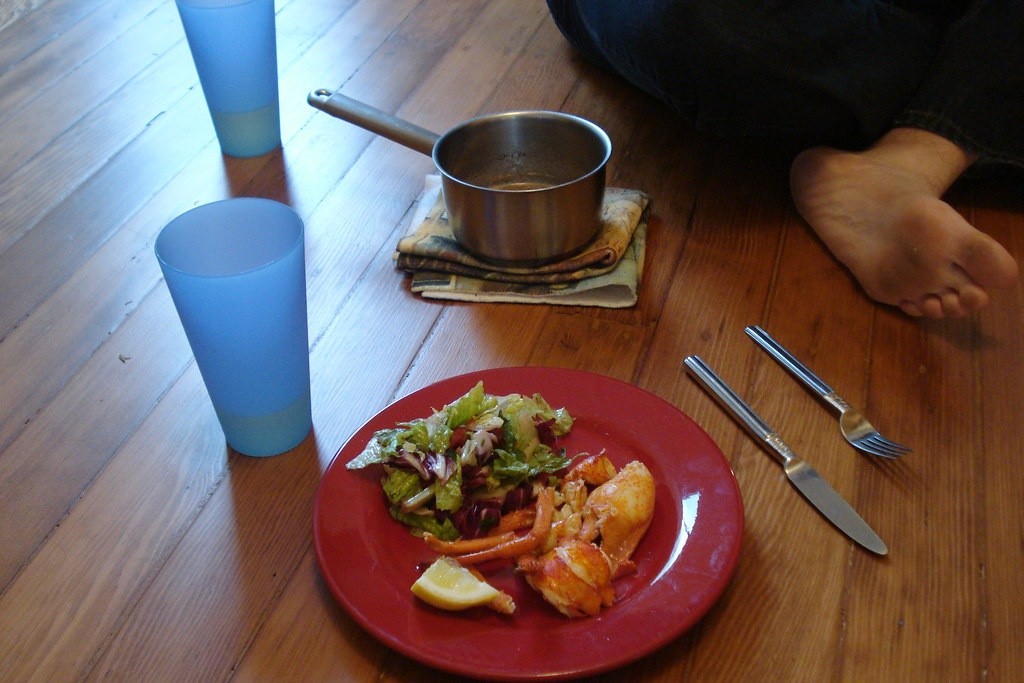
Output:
[345,379,584,536]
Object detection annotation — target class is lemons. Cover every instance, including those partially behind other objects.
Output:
[409,556,498,610]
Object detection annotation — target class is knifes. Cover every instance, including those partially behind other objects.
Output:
[673,354,888,557]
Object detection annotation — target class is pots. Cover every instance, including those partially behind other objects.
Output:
[306,87,611,268]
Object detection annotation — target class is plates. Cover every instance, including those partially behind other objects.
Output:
[314,365,745,683]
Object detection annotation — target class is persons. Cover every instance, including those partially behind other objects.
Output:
[544,0,1024,323]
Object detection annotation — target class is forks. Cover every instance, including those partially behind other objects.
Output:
[743,324,913,462]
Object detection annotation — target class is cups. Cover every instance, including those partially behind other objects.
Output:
[154,197,312,457]
[173,0,281,159]
